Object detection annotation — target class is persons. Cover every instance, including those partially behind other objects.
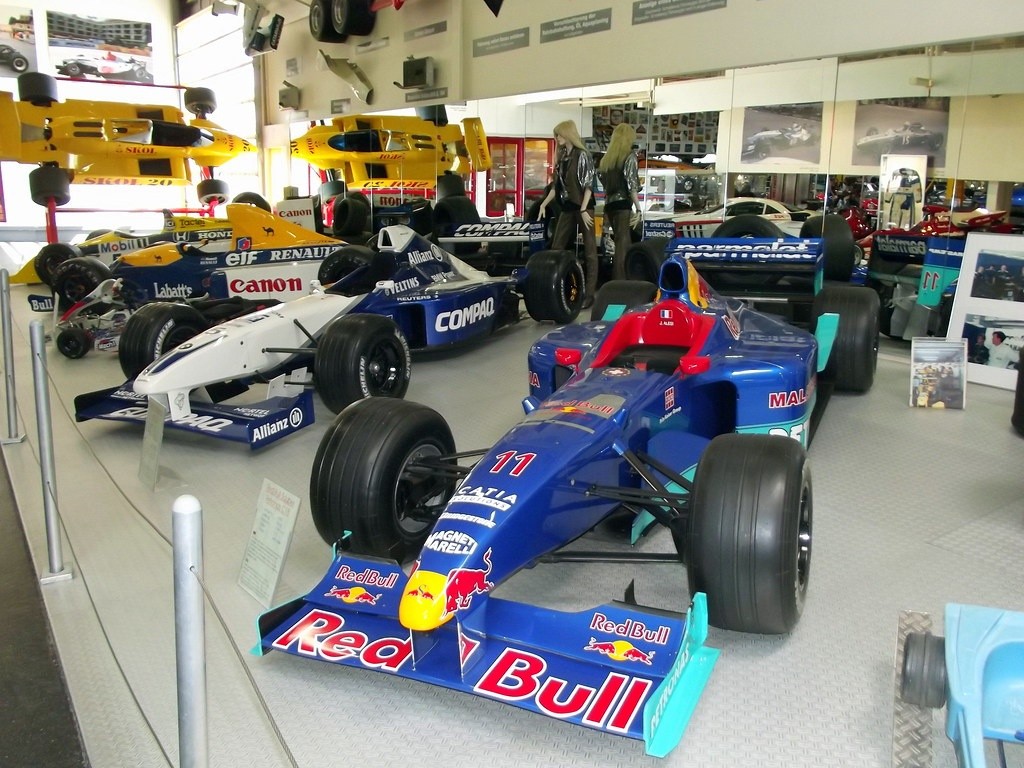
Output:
[537,119,642,309]
[912,265,1024,409]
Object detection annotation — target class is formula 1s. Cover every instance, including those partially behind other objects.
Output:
[287,104,491,207]
[10,174,1024,458]
[856,119,944,160]
[309,0,505,39]
[742,123,819,160]
[249,239,886,758]
[0,71,263,206]
[896,602,1024,768]
[0,42,29,71]
[56,51,155,83]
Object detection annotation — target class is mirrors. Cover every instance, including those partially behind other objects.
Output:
[262,30,1024,260]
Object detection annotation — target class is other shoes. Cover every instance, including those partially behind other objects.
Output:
[578,295,594,309]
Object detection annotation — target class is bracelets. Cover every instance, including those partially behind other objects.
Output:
[636,209,641,213]
[580,210,586,213]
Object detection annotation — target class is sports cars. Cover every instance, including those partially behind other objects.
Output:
[674,194,821,235]
